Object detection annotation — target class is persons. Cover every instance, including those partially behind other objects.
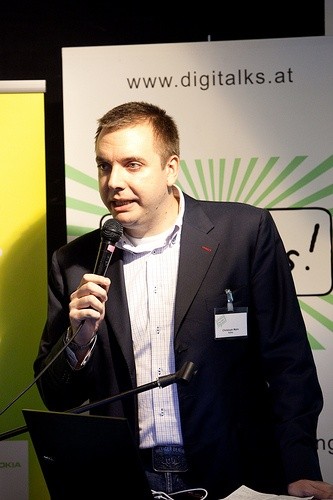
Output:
[33,102,333,500]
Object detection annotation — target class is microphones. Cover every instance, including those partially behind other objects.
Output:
[86,219,123,311]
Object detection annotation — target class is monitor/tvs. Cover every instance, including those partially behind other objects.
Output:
[21,409,156,500]
